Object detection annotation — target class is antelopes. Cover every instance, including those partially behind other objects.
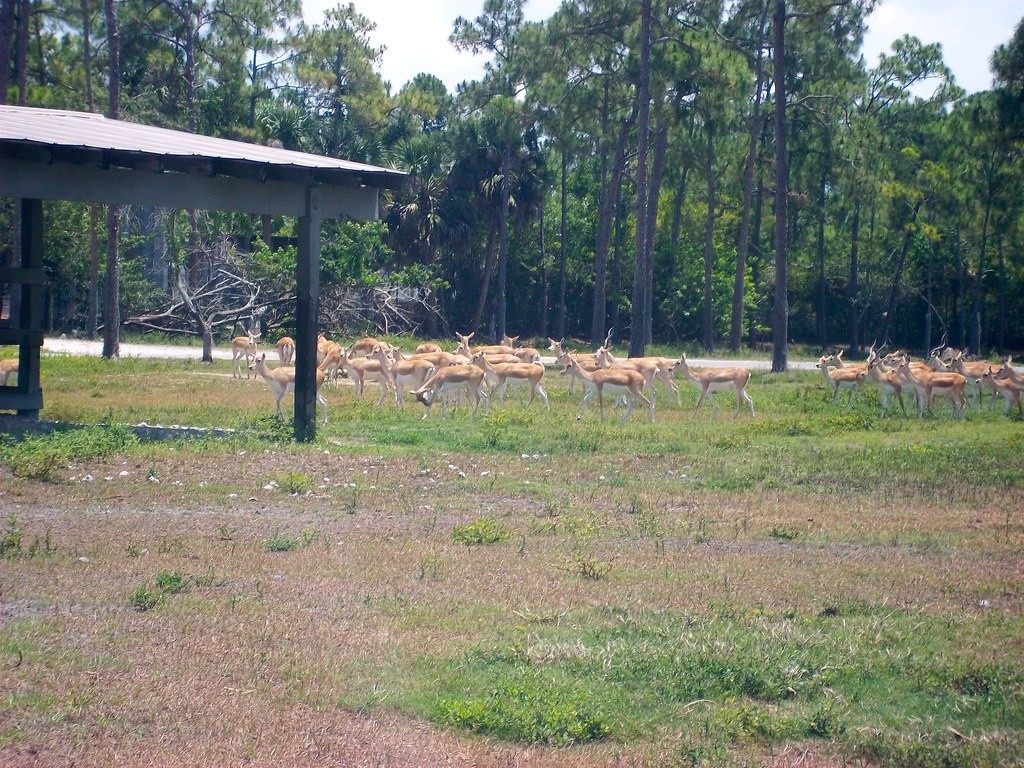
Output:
[225,326,1024,435]
